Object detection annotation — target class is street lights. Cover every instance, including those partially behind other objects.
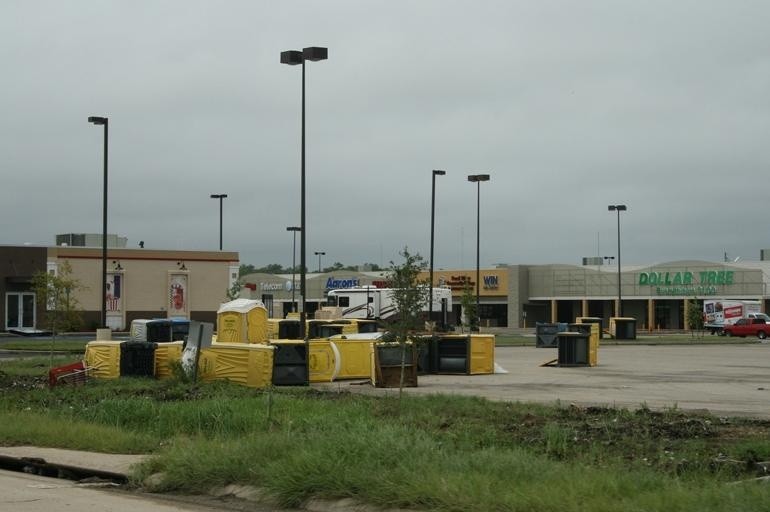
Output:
[429,170,445,285]
[280,47,327,312]
[608,205,627,315]
[88,116,108,328]
[468,174,490,324]
[210,194,227,250]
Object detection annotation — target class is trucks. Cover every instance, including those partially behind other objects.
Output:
[327,285,453,323]
[704,299,770,336]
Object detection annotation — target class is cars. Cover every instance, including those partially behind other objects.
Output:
[721,313,770,339]
